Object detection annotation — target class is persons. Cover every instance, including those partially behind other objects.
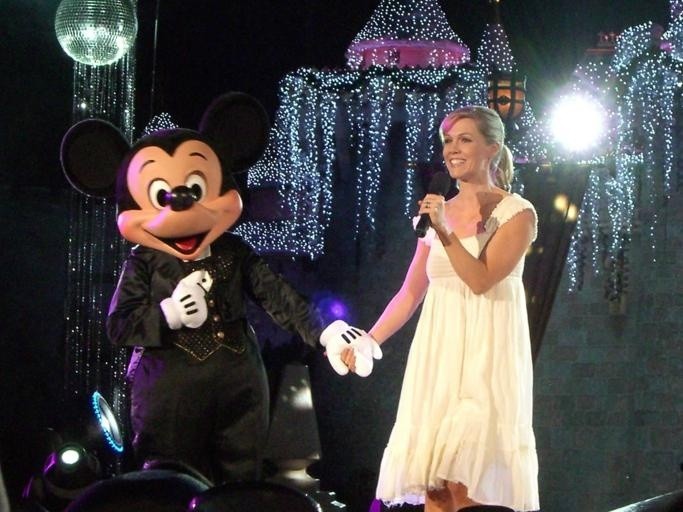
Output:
[340,106,540,512]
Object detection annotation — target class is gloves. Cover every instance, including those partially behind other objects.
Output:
[159,271,213,330]
[319,320,383,378]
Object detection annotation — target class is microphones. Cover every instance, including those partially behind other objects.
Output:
[415,172,452,238]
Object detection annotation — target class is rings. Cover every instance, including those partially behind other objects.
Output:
[425,202,430,208]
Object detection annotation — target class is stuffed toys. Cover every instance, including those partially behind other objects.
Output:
[61,92,383,484]
